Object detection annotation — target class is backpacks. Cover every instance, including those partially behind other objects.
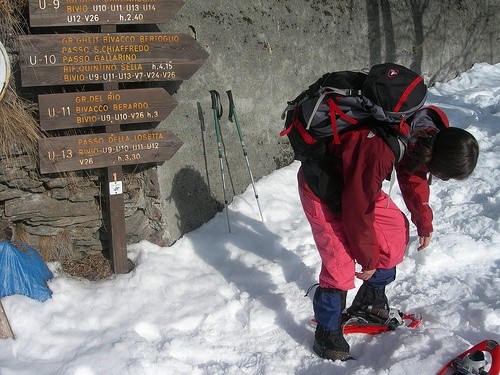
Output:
[279,62,447,180]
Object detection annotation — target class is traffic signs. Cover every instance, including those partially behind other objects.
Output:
[36,87,180,131]
[36,128,185,174]
[18,31,210,88]
[27,0,189,28]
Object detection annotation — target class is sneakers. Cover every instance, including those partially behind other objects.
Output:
[346,285,404,326]
[451,350,484,375]
[313,322,350,361]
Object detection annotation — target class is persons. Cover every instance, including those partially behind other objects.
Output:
[297,105,480,362]
